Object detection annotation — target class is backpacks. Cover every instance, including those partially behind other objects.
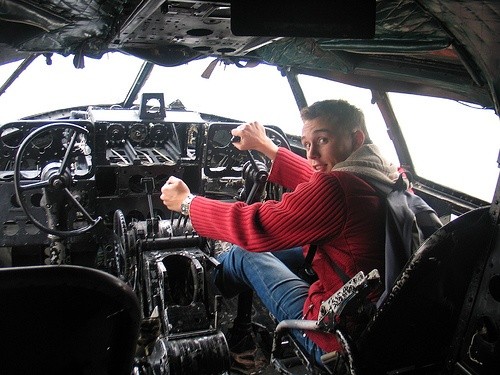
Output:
[320,172,444,309]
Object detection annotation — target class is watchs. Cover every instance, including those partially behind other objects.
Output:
[180,194,196,216]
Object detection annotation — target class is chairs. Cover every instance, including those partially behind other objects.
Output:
[0,266,141,375]
[266,203,499,375]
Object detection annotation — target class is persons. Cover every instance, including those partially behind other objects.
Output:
[159,99,414,365]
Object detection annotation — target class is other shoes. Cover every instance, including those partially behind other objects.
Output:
[205,264,222,299]
